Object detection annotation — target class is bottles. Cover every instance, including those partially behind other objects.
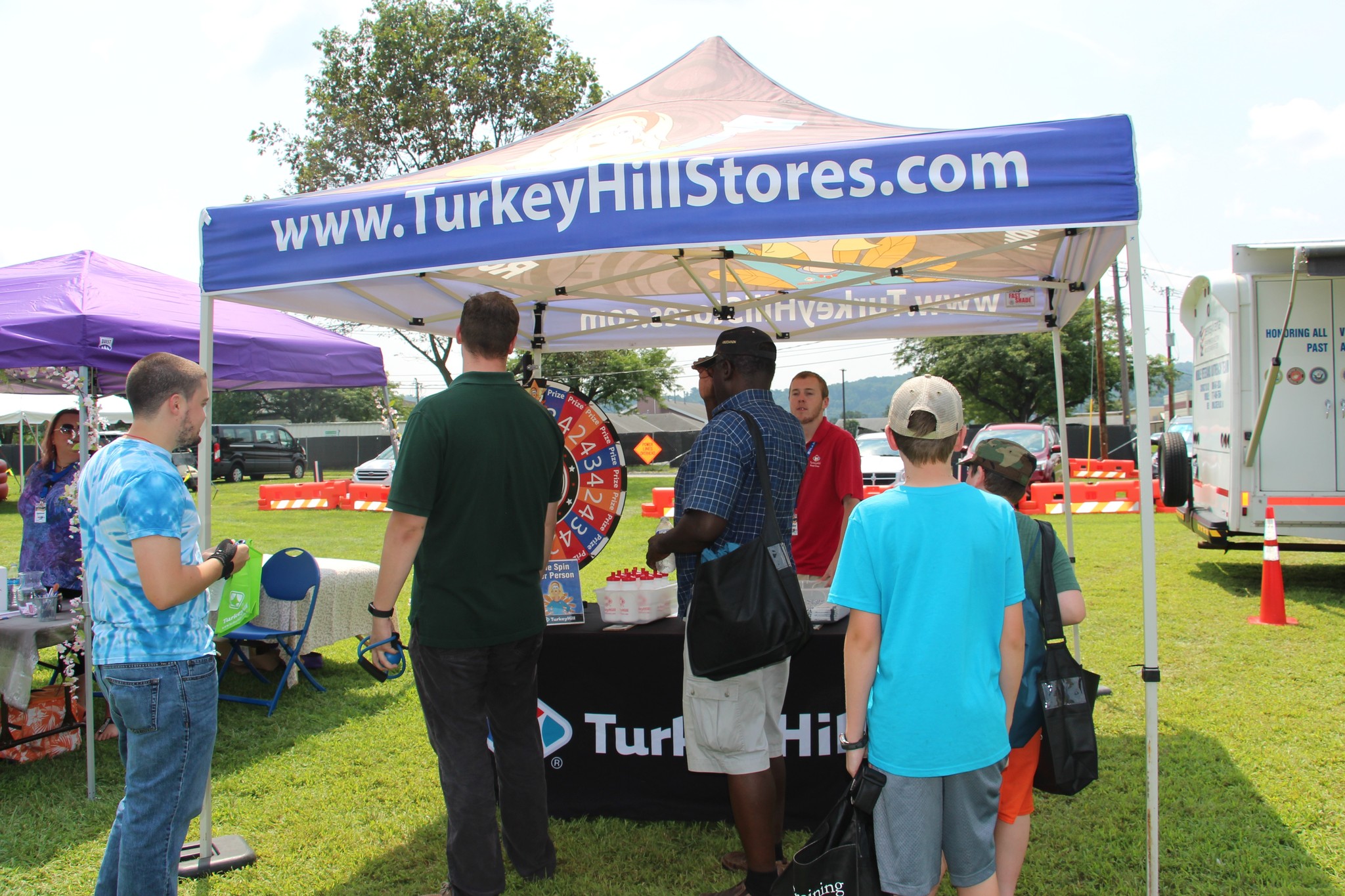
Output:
[17,571,48,618]
[7,563,22,610]
[656,516,677,575]
[606,567,672,622]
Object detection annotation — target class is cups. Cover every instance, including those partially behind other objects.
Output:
[35,595,58,621]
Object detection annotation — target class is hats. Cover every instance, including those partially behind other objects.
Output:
[888,374,965,439]
[695,326,776,368]
[956,438,1037,487]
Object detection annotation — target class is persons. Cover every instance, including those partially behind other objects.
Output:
[646,326,810,896]
[787,370,865,588]
[927,437,1086,896]
[77,351,251,896]
[371,291,566,896]
[826,373,1030,896]
[17,408,121,742]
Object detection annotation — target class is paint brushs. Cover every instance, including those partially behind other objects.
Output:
[49,583,59,598]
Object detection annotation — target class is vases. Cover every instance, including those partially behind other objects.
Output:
[15,571,47,618]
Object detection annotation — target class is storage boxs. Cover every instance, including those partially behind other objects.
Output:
[593,581,678,625]
[211,636,280,673]
[798,580,851,624]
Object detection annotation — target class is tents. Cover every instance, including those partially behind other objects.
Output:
[186,37,1161,896]
[0,251,400,798]
[0,392,134,498]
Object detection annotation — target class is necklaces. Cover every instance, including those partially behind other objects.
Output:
[124,433,154,445]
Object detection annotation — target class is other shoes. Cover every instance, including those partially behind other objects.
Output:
[721,850,788,877]
[699,879,750,896]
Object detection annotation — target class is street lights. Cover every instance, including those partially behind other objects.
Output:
[839,368,847,431]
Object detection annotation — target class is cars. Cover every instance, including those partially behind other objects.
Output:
[351,444,396,486]
[855,432,907,501]
[1151,415,1193,479]
[960,421,1062,500]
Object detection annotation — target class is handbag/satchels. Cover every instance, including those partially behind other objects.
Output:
[773,763,891,896]
[685,527,815,681]
[1032,641,1101,796]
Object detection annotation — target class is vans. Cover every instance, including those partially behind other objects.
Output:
[212,423,309,483]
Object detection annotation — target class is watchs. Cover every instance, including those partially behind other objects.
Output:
[839,730,869,750]
[368,602,396,618]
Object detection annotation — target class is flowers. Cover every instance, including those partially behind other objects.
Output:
[0,364,111,700]
[371,386,402,472]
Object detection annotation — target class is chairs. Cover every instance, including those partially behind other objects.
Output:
[214,548,328,718]
[260,433,270,442]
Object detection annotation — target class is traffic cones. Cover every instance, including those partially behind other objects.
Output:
[1246,506,1300,626]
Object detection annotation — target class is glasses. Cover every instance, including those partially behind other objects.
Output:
[53,423,79,435]
[705,359,723,378]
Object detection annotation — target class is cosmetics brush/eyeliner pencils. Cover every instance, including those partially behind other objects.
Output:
[37,583,60,621]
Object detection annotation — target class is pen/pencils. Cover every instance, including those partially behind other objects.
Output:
[35,583,59,622]
[0,616,10,621]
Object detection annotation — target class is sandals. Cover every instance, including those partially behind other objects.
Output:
[98,718,114,735]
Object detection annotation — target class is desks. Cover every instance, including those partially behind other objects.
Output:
[482,602,851,830]
[0,600,86,750]
[207,554,401,689]
